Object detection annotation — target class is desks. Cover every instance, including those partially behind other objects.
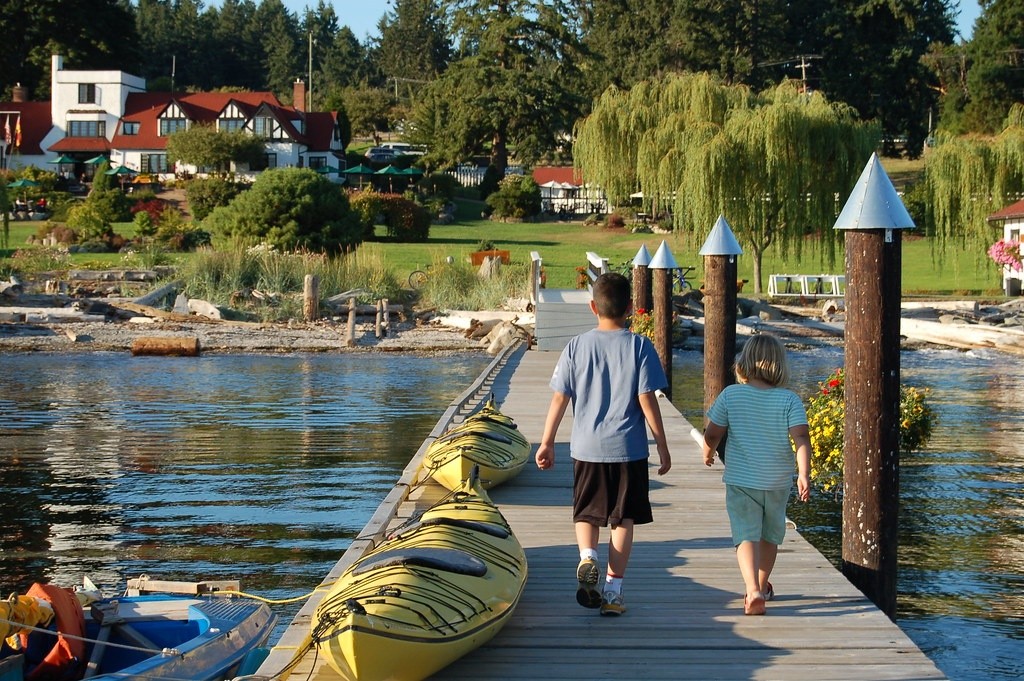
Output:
[638,213,649,222]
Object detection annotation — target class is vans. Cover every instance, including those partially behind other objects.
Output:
[364,147,407,157]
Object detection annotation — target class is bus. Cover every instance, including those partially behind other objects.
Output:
[380,143,428,156]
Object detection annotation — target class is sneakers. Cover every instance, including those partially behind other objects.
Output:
[761,581,774,600]
[574,554,603,609]
[599,587,627,615]
[743,591,767,616]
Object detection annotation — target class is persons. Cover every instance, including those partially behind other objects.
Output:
[535,272,671,615]
[15,197,46,213]
[56,171,133,194]
[705,334,814,617]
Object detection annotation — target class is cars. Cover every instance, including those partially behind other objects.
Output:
[366,152,398,162]
[12,192,72,213]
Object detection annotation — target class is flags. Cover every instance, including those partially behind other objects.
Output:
[4,115,22,146]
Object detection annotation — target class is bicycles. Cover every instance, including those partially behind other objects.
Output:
[407,263,438,291]
[542,200,607,223]
[607,262,695,296]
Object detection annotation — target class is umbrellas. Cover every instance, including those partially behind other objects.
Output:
[317,162,422,196]
[47,153,115,181]
[105,165,140,190]
[7,178,42,204]
[540,179,600,211]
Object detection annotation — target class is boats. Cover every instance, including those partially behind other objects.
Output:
[0,574,279,681]
[420,392,534,496]
[307,461,529,681]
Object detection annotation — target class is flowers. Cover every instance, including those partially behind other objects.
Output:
[987,239,1024,273]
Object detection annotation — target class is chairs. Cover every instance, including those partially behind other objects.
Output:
[10,199,36,214]
